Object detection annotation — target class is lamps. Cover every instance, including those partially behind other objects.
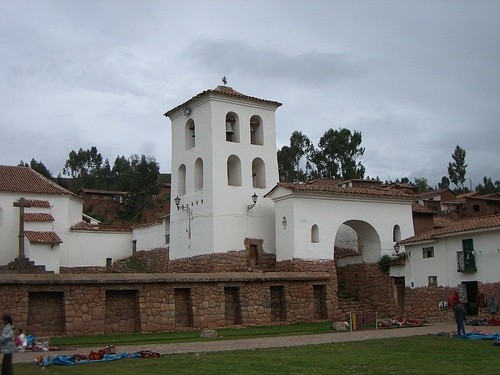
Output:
[247,192,258,212]
[393,243,400,254]
[175,196,190,212]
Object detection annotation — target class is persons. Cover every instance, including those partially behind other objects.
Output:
[454,301,466,335]
[0,315,15,375]
[17,328,27,348]
